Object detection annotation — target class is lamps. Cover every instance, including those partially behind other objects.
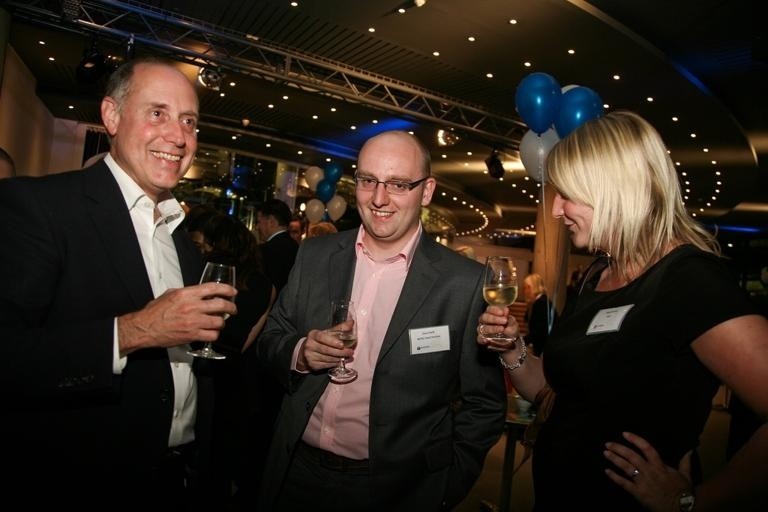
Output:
[483,147,509,181]
[437,118,461,148]
[194,52,228,91]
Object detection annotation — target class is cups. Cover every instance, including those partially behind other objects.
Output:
[515,395,532,413]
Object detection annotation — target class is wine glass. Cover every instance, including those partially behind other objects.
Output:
[482,257,519,342]
[187,262,237,360]
[327,299,359,383]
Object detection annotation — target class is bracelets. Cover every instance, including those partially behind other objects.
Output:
[678,488,697,511]
[498,336,527,371]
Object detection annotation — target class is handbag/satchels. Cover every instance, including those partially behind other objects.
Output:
[523,390,557,450]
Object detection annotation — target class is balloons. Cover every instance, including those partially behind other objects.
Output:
[509,72,600,190]
[300,160,346,227]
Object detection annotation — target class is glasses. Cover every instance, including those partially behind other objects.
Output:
[352,169,431,194]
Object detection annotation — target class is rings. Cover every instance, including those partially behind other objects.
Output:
[479,325,485,334]
[631,468,641,476]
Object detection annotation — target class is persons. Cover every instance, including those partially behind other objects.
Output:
[518,274,559,359]
[172,196,339,371]
[253,128,505,512]
[0,149,17,181]
[1,57,262,512]
[477,105,767,509]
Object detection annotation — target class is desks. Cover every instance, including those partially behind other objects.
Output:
[479,404,536,512]
